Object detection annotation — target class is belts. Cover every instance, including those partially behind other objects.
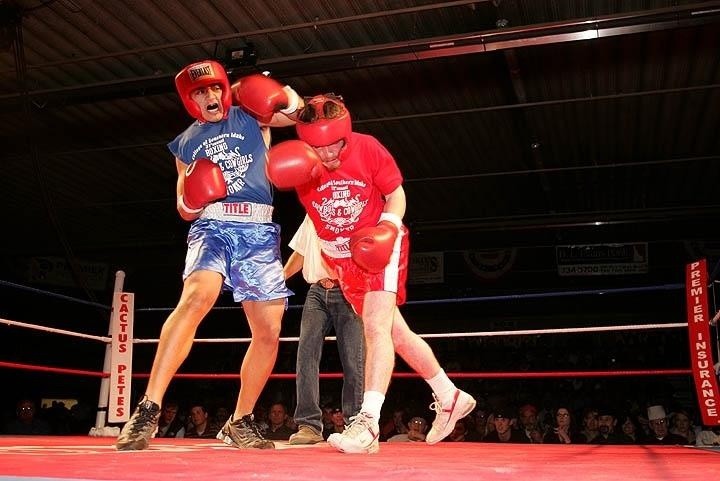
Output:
[316,278,339,289]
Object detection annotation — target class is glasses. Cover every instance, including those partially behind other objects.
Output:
[653,418,665,426]
[412,419,423,424]
[557,413,568,417]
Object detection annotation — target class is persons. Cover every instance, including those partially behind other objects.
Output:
[1,396,236,439]
[267,93,478,453]
[261,402,474,442]
[284,213,367,445]
[465,402,720,446]
[116,61,305,450]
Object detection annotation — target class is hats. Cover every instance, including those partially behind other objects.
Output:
[493,406,512,418]
[648,405,666,421]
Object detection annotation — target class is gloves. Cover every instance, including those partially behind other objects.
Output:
[264,140,323,189]
[232,74,288,124]
[179,160,226,212]
[350,213,403,274]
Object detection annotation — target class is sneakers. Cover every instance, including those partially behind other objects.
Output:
[217,412,275,448]
[116,395,161,450]
[426,389,477,445]
[327,411,380,453]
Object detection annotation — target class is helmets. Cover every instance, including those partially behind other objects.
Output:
[175,60,232,122]
[294,95,352,172]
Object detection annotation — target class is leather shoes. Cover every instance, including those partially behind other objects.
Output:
[289,426,325,444]
